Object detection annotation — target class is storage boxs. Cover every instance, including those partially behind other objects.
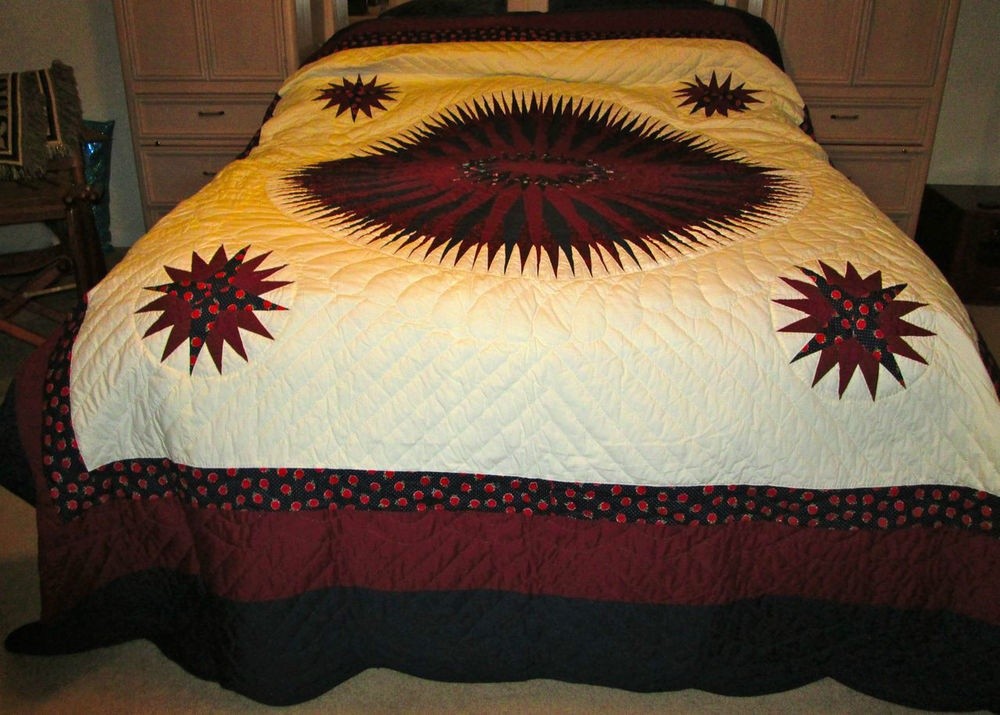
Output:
[916,185,1000,307]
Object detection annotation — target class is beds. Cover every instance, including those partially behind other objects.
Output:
[0,0,1000,715]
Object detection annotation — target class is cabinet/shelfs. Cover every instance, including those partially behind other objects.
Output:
[774,0,961,239]
[112,0,300,238]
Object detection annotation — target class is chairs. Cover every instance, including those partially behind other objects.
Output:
[1,60,106,301]
